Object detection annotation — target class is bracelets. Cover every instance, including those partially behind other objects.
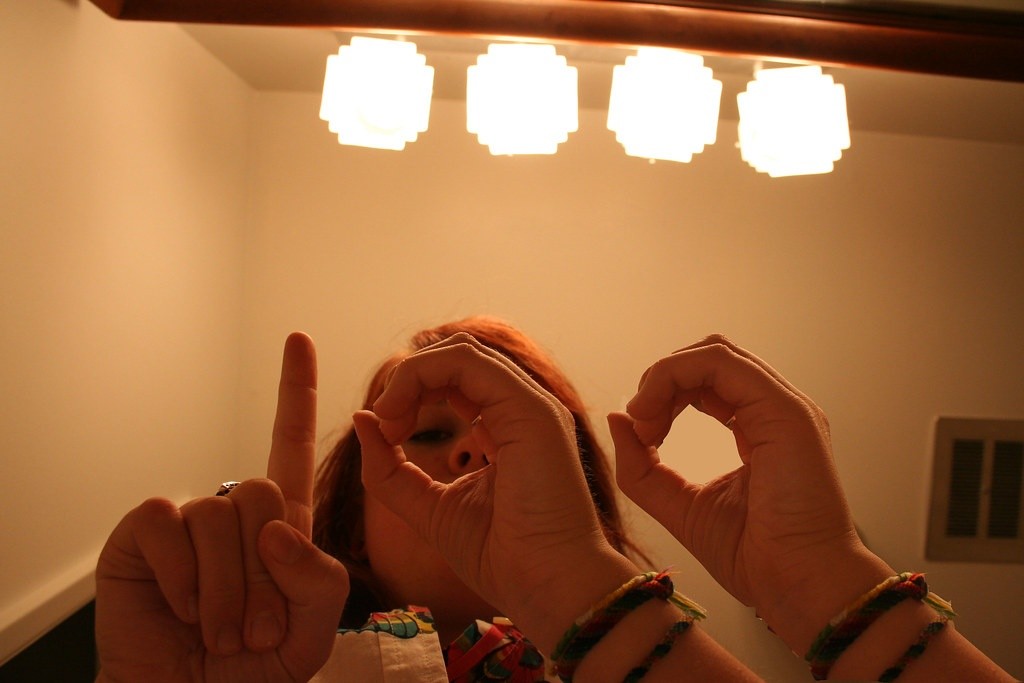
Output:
[550,571,707,683]
[804,572,955,683]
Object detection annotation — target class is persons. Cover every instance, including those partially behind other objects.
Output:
[607,335,1024,683]
[95,317,762,683]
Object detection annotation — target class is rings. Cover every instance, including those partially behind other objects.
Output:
[216,481,241,496]
